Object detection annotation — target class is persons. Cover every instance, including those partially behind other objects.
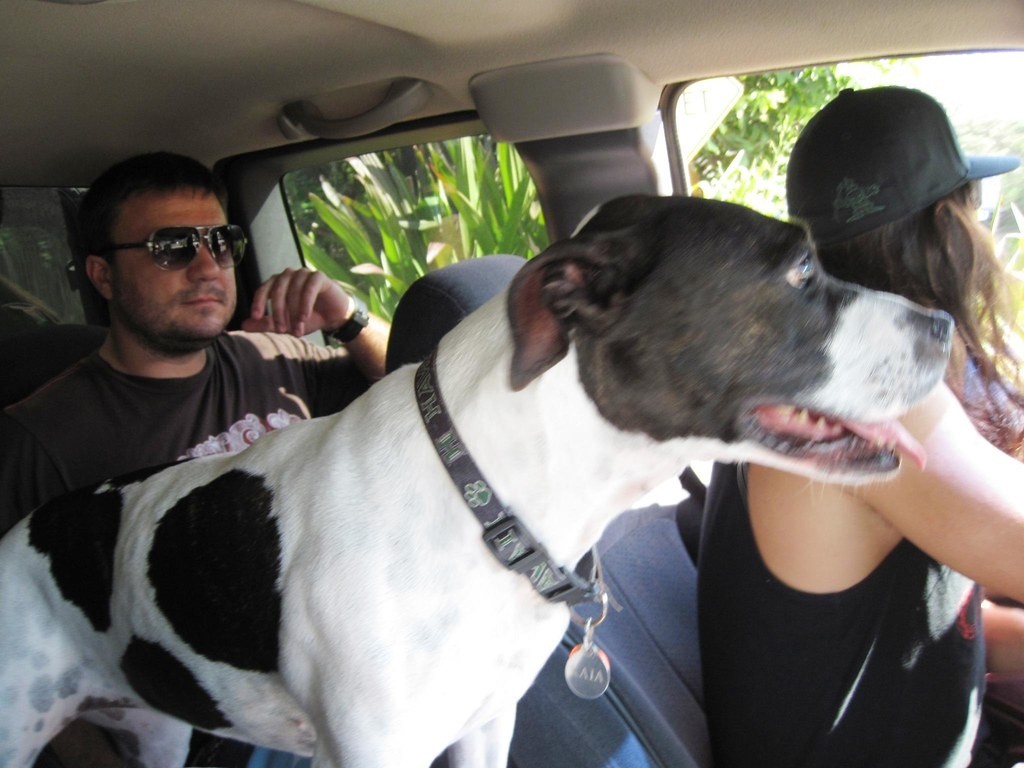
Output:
[1,153,387,768]
[699,85,1024,768]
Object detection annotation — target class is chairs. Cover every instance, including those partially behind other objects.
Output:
[385,254,713,768]
[0,324,110,409]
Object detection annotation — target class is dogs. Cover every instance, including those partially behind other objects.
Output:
[0,194,955,768]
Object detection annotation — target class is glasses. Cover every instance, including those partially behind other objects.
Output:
[100,224,247,271]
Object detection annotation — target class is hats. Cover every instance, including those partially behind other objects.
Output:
[786,87,1020,246]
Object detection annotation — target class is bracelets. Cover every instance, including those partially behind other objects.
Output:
[322,291,369,346]
